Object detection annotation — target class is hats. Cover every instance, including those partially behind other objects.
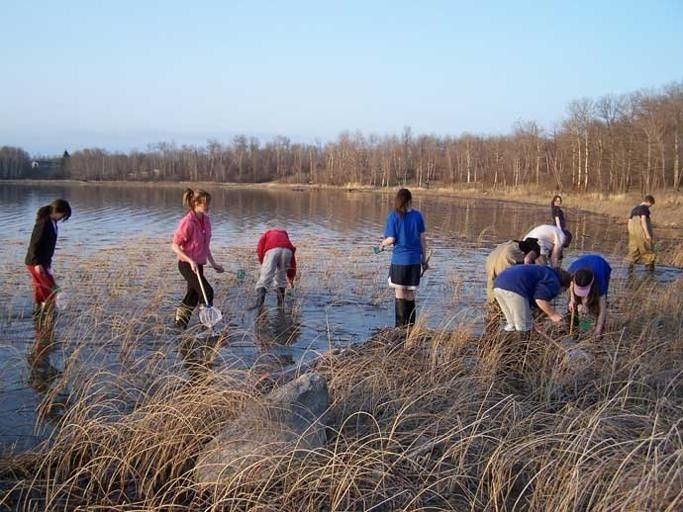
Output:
[574,272,594,297]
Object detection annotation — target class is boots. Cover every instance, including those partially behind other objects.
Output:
[248,288,266,310]
[277,287,285,305]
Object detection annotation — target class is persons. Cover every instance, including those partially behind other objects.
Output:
[378,188,428,326]
[491,264,571,333]
[484,237,540,306]
[246,228,296,314]
[566,253,611,344]
[177,331,227,387]
[23,198,71,312]
[171,186,225,330]
[254,310,301,372]
[550,195,566,260]
[521,224,572,268]
[26,312,69,423]
[622,195,656,271]
